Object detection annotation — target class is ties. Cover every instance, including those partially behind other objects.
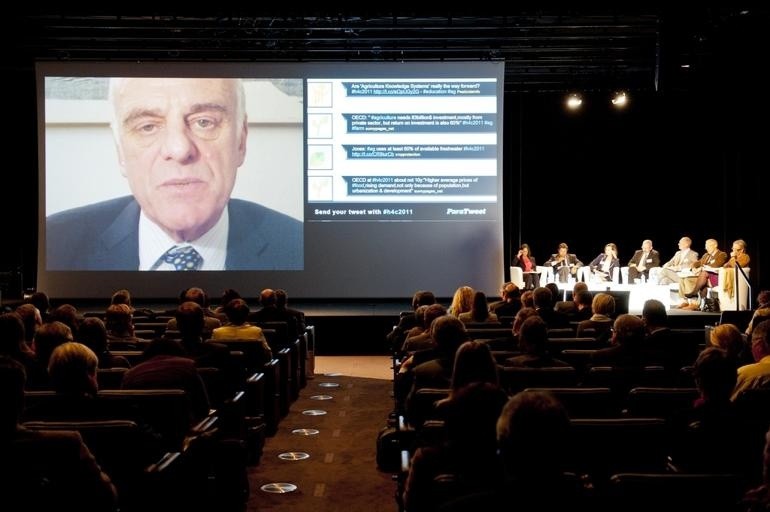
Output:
[163,248,202,271]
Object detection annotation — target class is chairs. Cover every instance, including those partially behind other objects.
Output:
[397,387,700,412]
[510,266,547,290]
[392,311,769,389]
[621,267,751,312]
[393,449,731,512]
[396,413,666,451]
[20,304,316,512]
[548,265,582,291]
[583,265,620,292]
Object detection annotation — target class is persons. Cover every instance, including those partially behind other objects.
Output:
[121,338,206,394]
[47,341,100,423]
[397,380,508,507]
[251,288,296,323]
[464,292,492,323]
[406,305,432,340]
[398,314,468,370]
[76,317,129,369]
[275,289,305,324]
[13,304,43,352]
[511,307,538,337]
[429,389,586,511]
[0,361,118,511]
[628,240,660,283]
[684,239,750,311]
[386,290,437,341]
[743,290,770,339]
[609,314,645,347]
[437,340,505,400]
[547,282,560,299]
[575,292,616,339]
[401,303,449,352]
[543,243,584,282]
[677,238,727,311]
[659,237,698,285]
[685,346,735,415]
[105,305,139,341]
[554,282,588,311]
[210,298,273,359]
[489,281,514,313]
[505,316,549,368]
[174,301,229,352]
[521,290,535,308]
[47,77,305,271]
[708,323,743,350]
[0,312,35,361]
[495,284,522,319]
[572,291,594,321]
[511,244,541,289]
[643,299,675,341]
[532,286,551,310]
[449,286,475,319]
[730,321,770,403]
[53,304,79,336]
[30,291,50,322]
[216,289,241,315]
[34,322,72,360]
[589,243,623,291]
[181,287,217,318]
[109,287,135,312]
